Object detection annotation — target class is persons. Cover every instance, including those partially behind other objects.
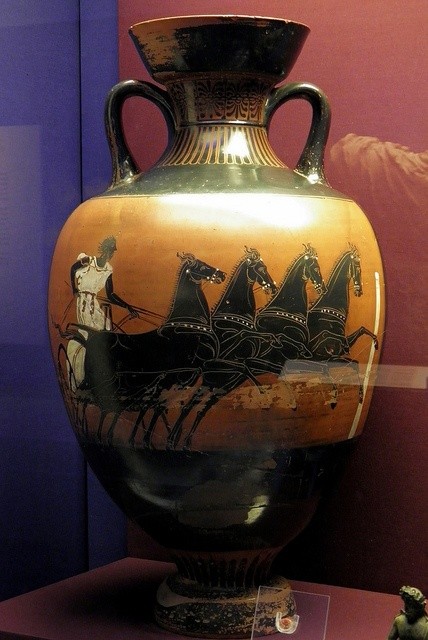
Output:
[66,236,140,392]
[389,586,427,640]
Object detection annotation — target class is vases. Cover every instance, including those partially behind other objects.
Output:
[47,16,384,637]
